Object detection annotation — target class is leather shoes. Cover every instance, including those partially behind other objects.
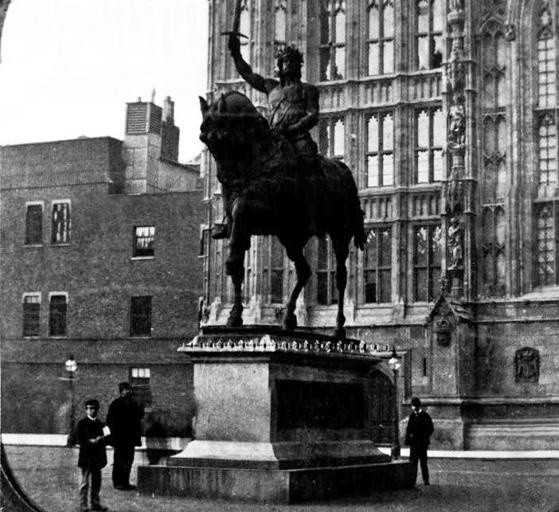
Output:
[128,483,137,489]
[115,484,129,490]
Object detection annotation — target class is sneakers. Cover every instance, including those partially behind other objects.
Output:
[424,481,429,485]
[92,506,107,512]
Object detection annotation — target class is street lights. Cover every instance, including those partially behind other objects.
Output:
[65,353,77,434]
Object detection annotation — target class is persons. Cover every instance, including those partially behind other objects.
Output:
[211,35,330,238]
[105,382,145,492]
[404,395,434,487]
[66,399,113,511]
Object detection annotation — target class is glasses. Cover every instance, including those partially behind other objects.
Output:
[84,407,95,410]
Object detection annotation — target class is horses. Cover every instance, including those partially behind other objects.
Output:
[199,91,367,339]
